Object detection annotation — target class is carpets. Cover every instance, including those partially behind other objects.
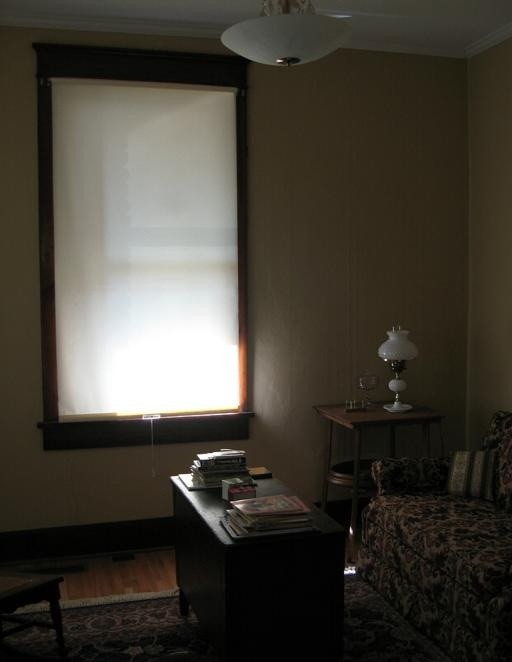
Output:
[0,576,465,660]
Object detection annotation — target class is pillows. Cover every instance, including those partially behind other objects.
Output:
[445,441,500,504]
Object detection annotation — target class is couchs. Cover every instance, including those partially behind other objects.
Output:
[357,405,512,661]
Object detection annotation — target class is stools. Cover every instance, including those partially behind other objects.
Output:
[0,570,70,659]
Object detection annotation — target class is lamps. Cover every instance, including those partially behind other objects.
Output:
[374,322,421,414]
[216,0,353,69]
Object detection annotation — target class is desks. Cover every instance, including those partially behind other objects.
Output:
[312,397,440,568]
[169,465,348,658]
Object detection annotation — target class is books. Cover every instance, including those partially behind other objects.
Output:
[178,448,321,540]
